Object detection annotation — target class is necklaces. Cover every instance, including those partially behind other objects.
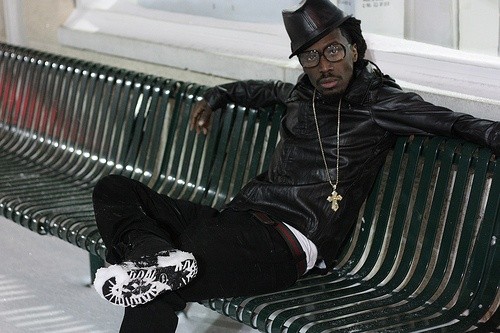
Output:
[312,88,343,211]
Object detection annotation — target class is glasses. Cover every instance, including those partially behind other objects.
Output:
[298,43,352,68]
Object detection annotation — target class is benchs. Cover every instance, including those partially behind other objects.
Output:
[0,40,500,333]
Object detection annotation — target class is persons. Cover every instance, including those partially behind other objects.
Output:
[93,0,500,333]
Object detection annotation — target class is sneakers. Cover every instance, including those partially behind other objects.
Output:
[93,237,199,308]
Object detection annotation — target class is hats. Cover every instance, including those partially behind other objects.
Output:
[282,0,352,60]
